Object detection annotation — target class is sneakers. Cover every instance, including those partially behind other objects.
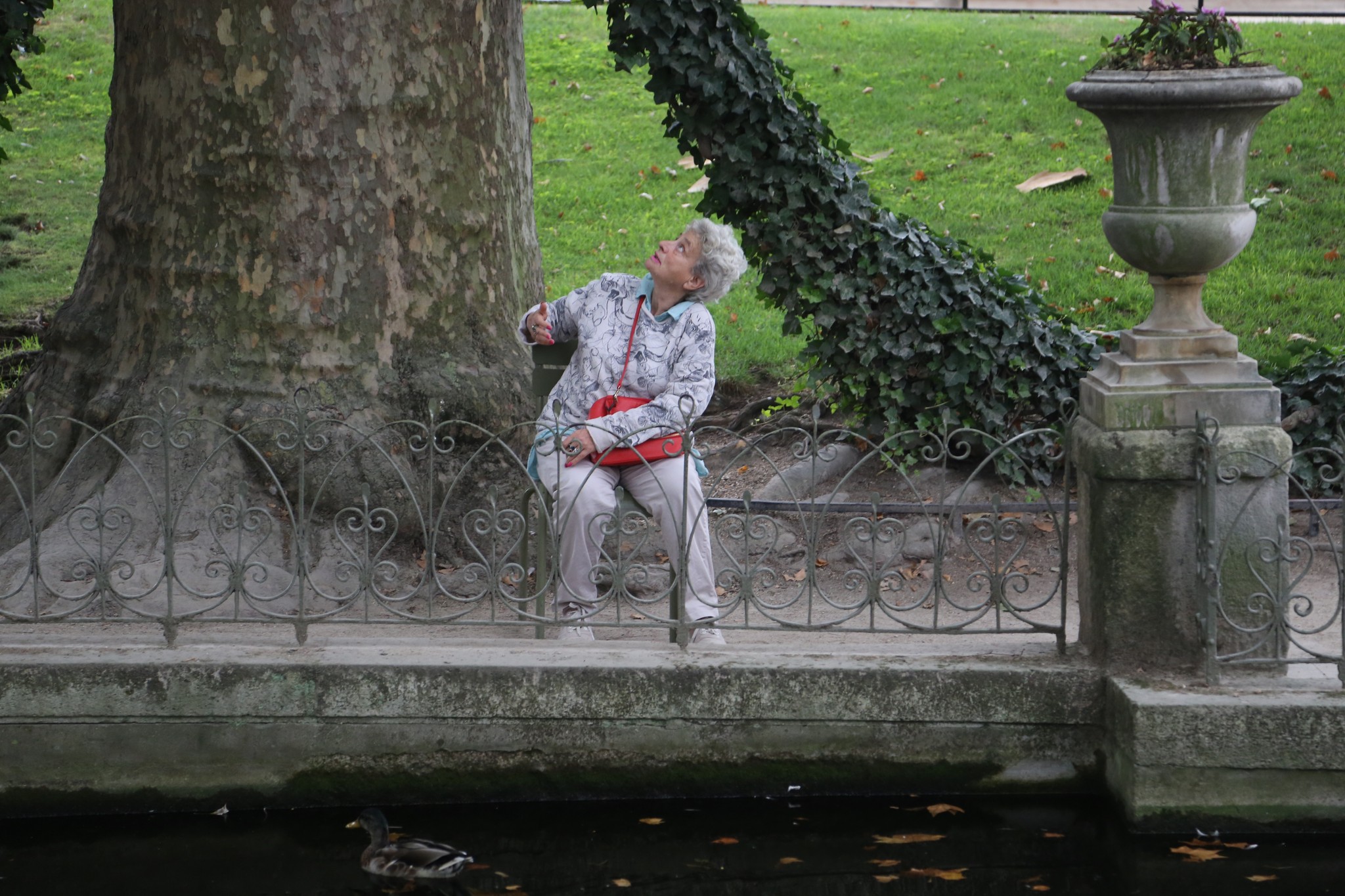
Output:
[689,618,727,644]
[557,602,595,641]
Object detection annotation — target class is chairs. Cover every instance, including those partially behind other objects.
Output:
[519,333,677,642]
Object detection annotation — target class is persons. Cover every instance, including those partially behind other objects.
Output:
[517,219,749,646]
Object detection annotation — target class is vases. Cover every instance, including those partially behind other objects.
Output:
[1065,70,1303,275]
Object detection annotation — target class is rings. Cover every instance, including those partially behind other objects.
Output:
[534,332,537,337]
[568,444,575,452]
[573,448,578,453]
[533,337,535,340]
[531,323,538,332]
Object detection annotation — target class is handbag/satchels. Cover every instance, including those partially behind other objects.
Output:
[585,394,687,465]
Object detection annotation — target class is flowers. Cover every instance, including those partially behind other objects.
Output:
[1084,0,1264,68]
